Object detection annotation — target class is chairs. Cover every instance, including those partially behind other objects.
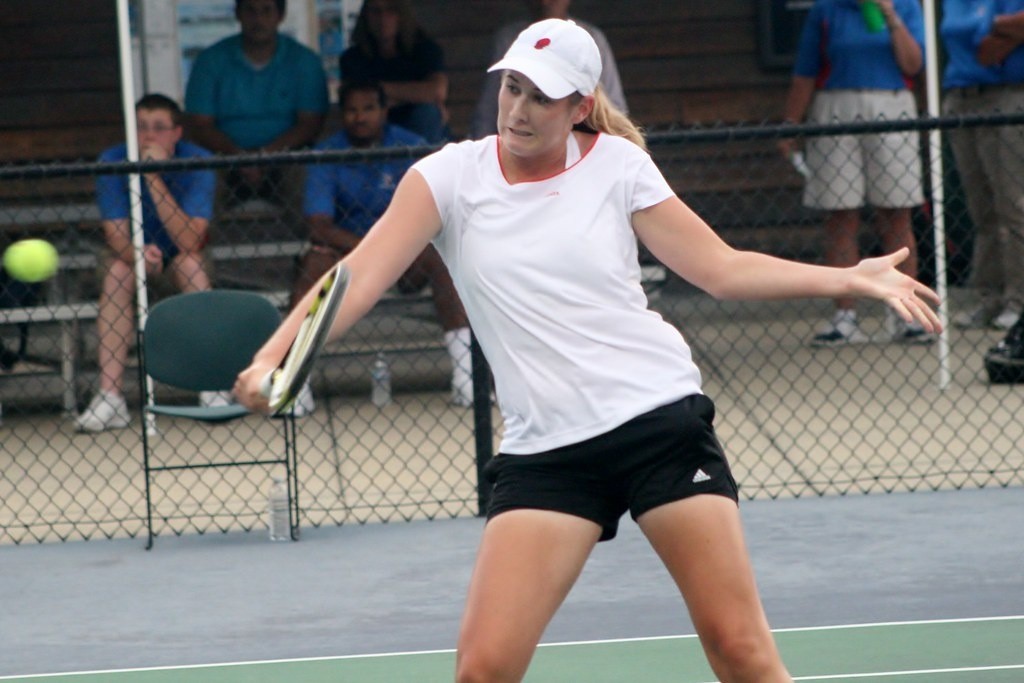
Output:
[135,288,300,552]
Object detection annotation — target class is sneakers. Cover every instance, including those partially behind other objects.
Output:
[198,386,231,408]
[450,365,495,407]
[273,377,316,418]
[72,392,130,433]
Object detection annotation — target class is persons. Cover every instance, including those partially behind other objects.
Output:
[269,77,496,415]
[473,0,627,138]
[940,0,1024,384]
[339,0,452,144]
[232,16,943,683]
[774,0,936,349]
[74,92,232,434]
[183,0,329,243]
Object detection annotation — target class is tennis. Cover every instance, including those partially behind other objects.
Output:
[2,237,61,285]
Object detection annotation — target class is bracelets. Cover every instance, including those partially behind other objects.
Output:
[890,20,901,30]
[781,119,793,124]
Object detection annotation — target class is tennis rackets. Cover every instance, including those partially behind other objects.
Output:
[265,262,352,422]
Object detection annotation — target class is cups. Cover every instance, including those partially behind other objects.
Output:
[858,0,887,34]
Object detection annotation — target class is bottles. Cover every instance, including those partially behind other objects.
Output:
[371,352,392,407]
[268,477,293,543]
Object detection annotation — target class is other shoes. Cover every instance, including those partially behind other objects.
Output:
[953,294,990,324]
[812,317,862,346]
[889,319,933,343]
[994,301,1021,329]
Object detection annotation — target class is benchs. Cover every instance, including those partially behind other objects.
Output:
[0,197,667,420]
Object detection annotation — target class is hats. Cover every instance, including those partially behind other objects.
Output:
[487,17,603,99]
